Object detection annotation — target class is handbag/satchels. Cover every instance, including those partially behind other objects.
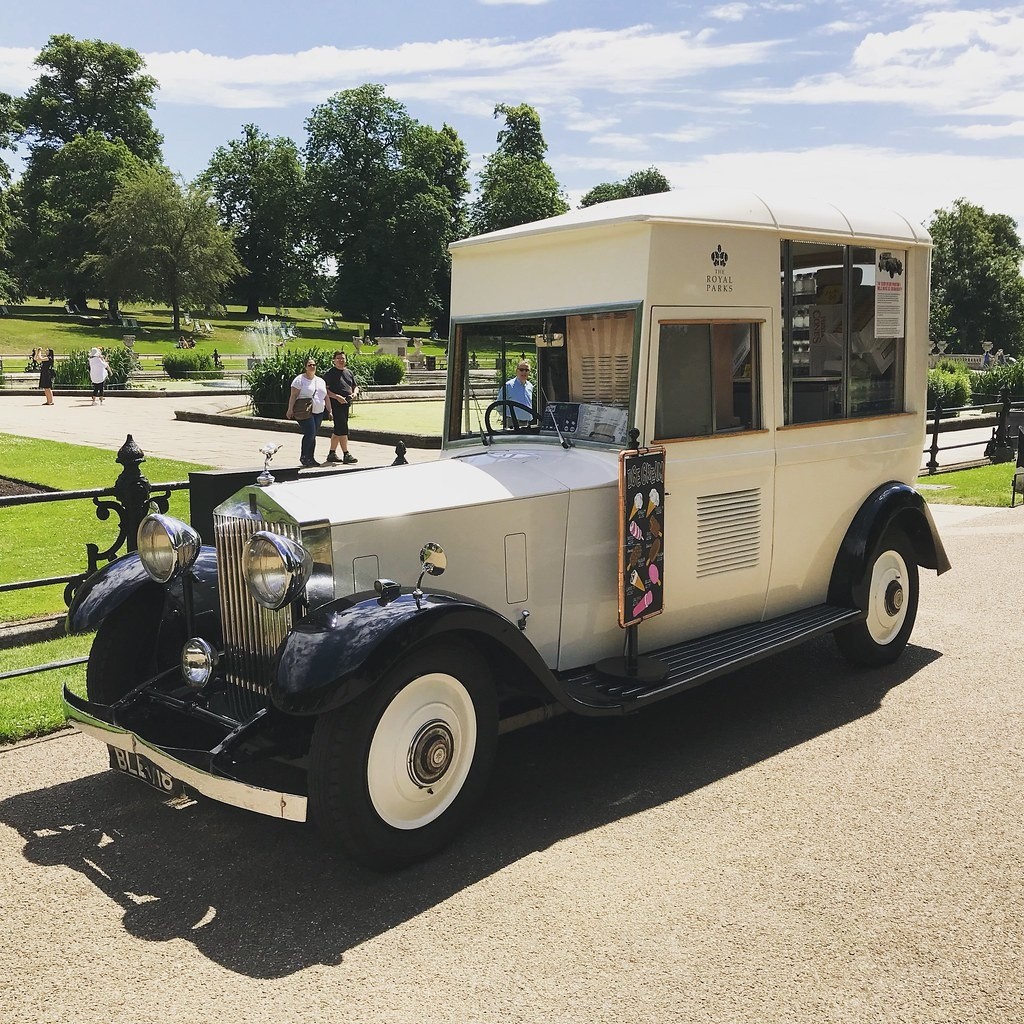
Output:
[293,377,317,420]
[49,369,56,378]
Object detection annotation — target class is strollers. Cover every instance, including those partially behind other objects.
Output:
[25,349,43,372]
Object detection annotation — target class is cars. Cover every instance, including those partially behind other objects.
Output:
[58,182,954,879]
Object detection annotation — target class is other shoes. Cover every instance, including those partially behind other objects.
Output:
[92,402,98,405]
[300,457,320,466]
[100,402,105,405]
[42,403,54,405]
[328,453,342,462]
[343,453,358,464]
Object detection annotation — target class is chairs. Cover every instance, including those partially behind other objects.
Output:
[279,322,302,343]
[171,313,182,327]
[276,307,290,317]
[191,320,203,335]
[64,305,74,314]
[183,312,193,326]
[1012,426,1024,506]
[1,306,9,315]
[105,310,139,347]
[322,318,338,330]
[203,322,215,333]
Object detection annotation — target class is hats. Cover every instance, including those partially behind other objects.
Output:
[88,348,102,356]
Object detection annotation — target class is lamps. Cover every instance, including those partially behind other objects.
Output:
[353,336,364,353]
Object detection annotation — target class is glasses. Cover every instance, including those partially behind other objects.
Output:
[517,368,529,372]
[308,364,316,366]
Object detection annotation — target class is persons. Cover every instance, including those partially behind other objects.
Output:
[88,346,113,406]
[984,351,991,366]
[383,302,403,337]
[432,330,438,339]
[29,347,54,405]
[496,361,534,428]
[286,351,359,467]
[178,336,195,347]
